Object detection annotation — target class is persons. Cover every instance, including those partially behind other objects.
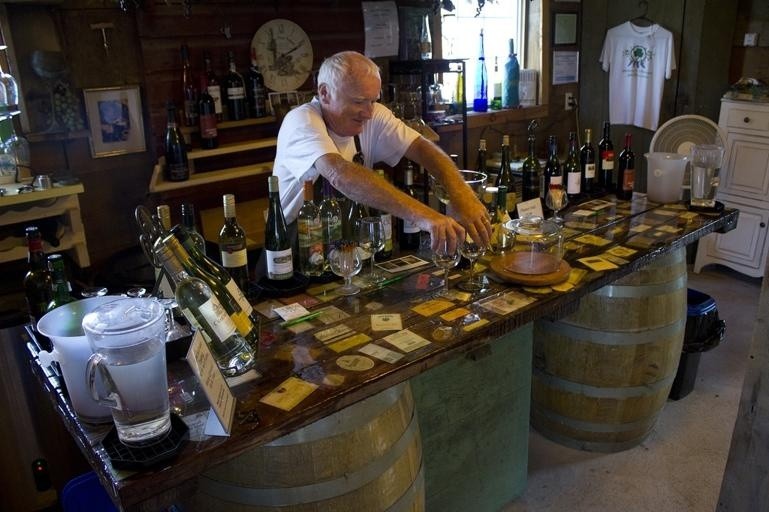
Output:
[254,51,491,282]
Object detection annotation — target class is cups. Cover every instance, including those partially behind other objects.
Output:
[39,297,130,425]
[81,297,173,450]
[688,143,726,208]
[644,150,690,205]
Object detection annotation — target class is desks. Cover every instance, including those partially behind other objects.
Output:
[0,173,90,269]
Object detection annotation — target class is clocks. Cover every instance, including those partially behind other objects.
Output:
[250,19,314,93]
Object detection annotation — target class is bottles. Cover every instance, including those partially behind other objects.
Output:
[35,220,60,248]
[162,105,191,182]
[297,179,325,279]
[597,121,615,192]
[38,174,52,190]
[616,132,636,201]
[389,73,447,124]
[581,128,597,197]
[493,133,517,221]
[453,31,521,115]
[133,175,297,379]
[396,160,422,251]
[21,225,54,339]
[42,253,82,337]
[408,13,433,62]
[564,130,583,207]
[319,180,343,272]
[522,134,542,203]
[543,134,563,188]
[177,41,268,150]
[367,169,393,262]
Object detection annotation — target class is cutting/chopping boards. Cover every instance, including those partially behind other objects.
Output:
[489,250,572,286]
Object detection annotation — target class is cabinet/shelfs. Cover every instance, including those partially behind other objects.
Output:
[377,56,468,240]
[147,114,285,254]
[694,98,769,277]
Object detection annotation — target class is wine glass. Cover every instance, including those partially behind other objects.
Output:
[545,188,569,222]
[328,242,364,296]
[430,231,485,300]
[358,216,387,283]
[0,45,33,179]
[33,50,73,134]
[79,284,191,345]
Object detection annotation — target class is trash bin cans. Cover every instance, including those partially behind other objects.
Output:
[667,287,717,401]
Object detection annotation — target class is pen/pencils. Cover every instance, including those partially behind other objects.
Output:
[278,309,323,328]
[381,272,412,285]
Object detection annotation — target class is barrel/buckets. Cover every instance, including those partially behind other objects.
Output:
[196,379,426,512]
[530,245,687,454]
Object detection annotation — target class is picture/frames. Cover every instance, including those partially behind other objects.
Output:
[553,10,580,48]
[552,50,580,85]
[80,85,148,160]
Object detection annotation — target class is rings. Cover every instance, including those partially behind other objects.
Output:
[483,219,490,225]
